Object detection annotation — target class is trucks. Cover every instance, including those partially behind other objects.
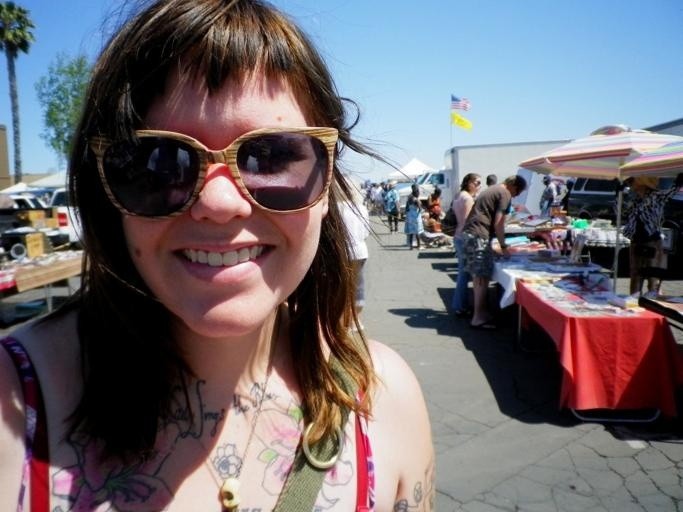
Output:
[391,139,574,215]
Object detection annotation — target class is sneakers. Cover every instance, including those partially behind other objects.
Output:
[455,304,473,318]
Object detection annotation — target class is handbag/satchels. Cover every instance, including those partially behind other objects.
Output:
[440,209,457,236]
[634,244,656,258]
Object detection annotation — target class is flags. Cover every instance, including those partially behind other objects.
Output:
[450,94,472,109]
[449,110,471,132]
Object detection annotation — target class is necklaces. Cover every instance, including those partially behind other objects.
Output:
[152,301,282,510]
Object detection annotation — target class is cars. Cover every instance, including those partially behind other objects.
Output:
[0,188,84,266]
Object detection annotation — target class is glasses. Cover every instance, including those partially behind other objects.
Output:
[87,127,338,220]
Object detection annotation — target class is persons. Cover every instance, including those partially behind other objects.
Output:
[620,175,682,294]
[335,171,366,333]
[559,179,574,214]
[537,173,557,217]
[0,1,432,511]
[485,174,498,187]
[360,178,442,250]
[451,170,484,316]
[460,175,527,326]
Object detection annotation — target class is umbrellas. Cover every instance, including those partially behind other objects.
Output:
[616,135,682,179]
[519,122,682,294]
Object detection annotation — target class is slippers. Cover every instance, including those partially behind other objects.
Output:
[469,321,497,330]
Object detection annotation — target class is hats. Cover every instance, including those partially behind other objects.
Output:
[636,176,660,190]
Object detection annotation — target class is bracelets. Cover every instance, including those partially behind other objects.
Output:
[500,244,509,248]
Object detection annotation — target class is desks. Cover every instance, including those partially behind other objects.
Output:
[491,245,615,329]
[515,279,682,423]
